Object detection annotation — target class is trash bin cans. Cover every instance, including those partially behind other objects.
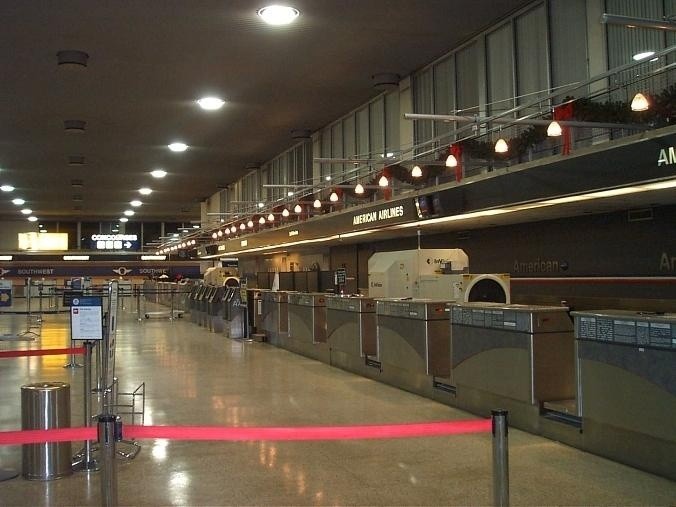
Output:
[20,382,72,482]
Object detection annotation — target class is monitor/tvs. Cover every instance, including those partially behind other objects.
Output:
[417,195,435,216]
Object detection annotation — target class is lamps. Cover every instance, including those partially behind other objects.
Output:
[211,214,275,238]
[379,170,388,186]
[313,192,321,208]
[631,92,648,111]
[281,204,290,217]
[355,178,364,193]
[294,200,301,213]
[495,131,508,152]
[411,157,422,176]
[446,145,457,167]
[330,186,338,202]
[547,110,562,136]
